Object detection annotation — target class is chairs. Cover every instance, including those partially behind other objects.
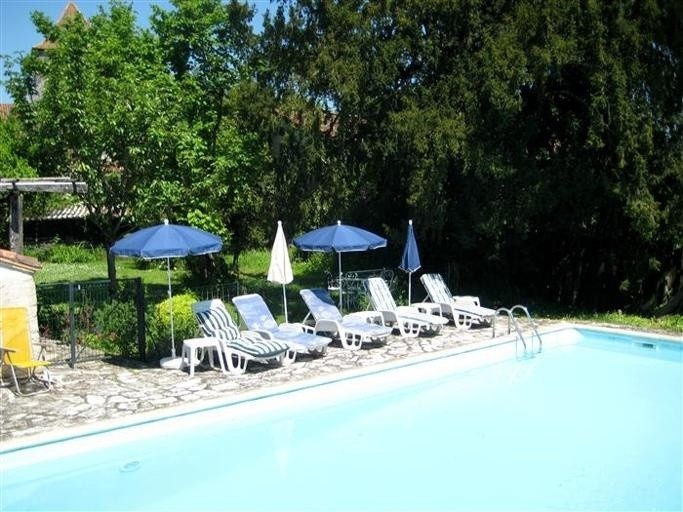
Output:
[1,306,54,397]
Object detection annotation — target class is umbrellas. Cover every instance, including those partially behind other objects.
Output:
[109,218,224,370]
[266,219,294,322]
[397,219,423,306]
[292,219,387,315]
